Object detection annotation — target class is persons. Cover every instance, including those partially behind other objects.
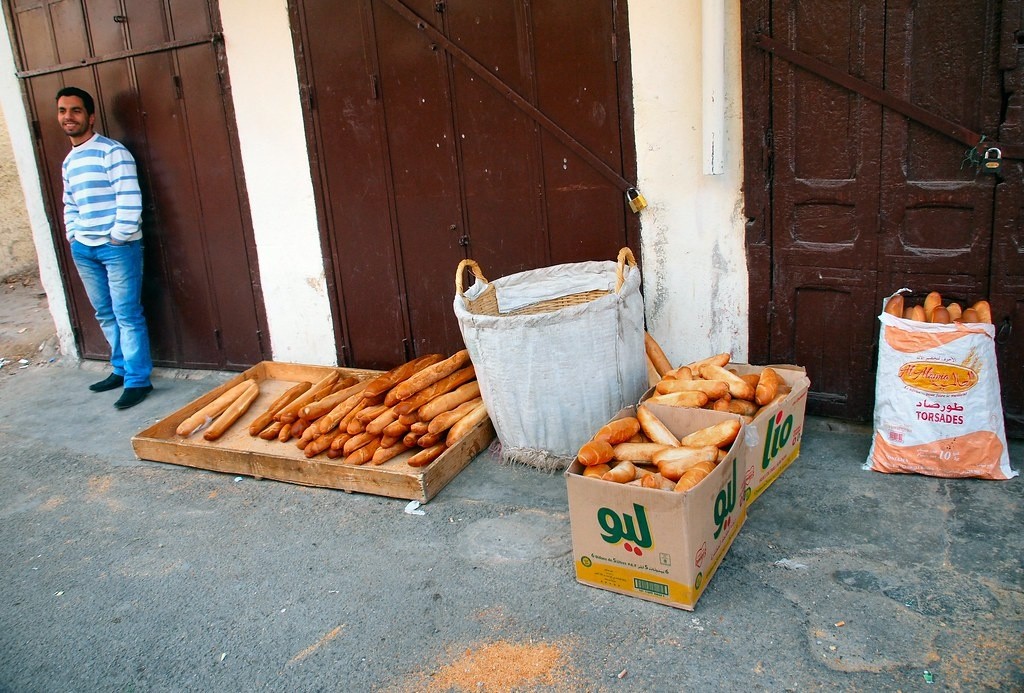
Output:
[55,86,154,410]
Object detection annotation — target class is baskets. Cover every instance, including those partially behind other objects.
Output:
[453,246,649,470]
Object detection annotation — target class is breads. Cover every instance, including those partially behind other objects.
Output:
[885,291,991,324]
[249,348,488,467]
[643,332,792,425]
[176,378,259,441]
[579,404,742,494]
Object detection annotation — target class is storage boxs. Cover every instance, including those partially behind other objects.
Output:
[563,402,747,612]
[639,361,811,508]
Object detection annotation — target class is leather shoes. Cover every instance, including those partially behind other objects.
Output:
[114,384,153,409]
[89,373,124,393]
